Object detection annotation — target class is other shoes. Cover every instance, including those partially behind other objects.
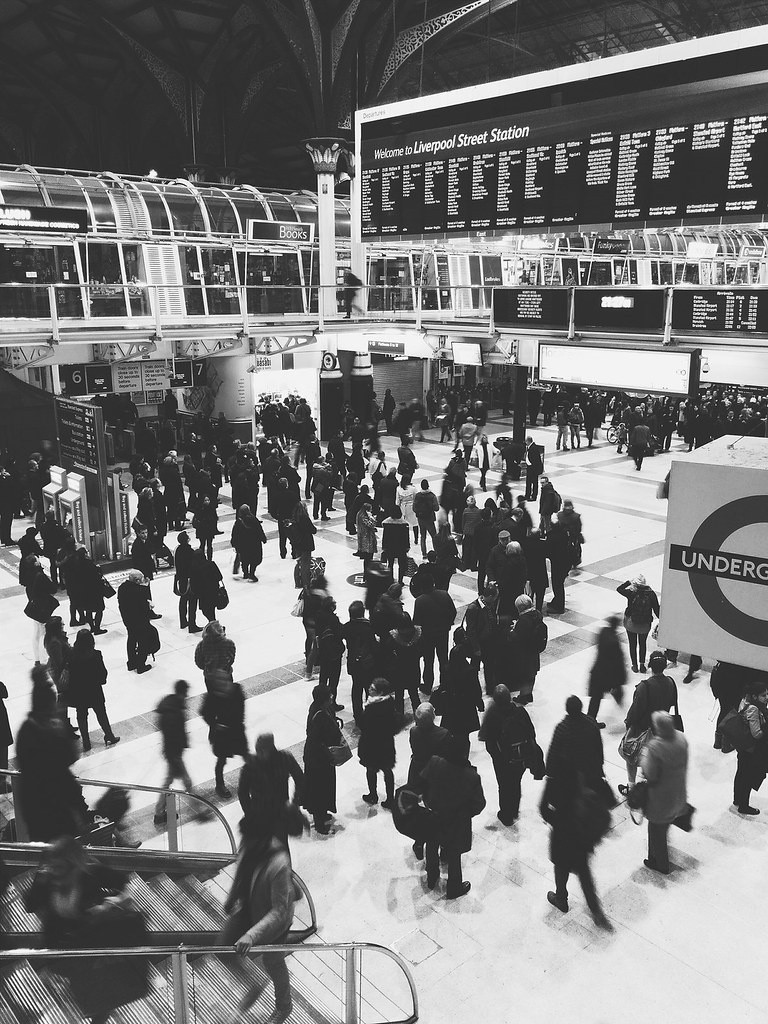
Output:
[137,664,152,674]
[189,626,203,634]
[363,793,378,806]
[180,621,189,629]
[128,664,138,671]
[382,799,394,810]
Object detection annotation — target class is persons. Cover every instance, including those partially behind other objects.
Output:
[0,376,768,1024]
[341,267,363,319]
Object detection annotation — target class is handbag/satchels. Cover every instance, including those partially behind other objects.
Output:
[405,557,417,577]
[717,707,757,753]
[192,512,201,529]
[326,740,354,767]
[671,705,684,732]
[218,586,229,610]
[453,626,467,648]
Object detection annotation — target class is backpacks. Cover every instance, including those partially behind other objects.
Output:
[144,622,161,661]
[351,637,386,672]
[429,688,451,716]
[492,735,531,779]
[392,782,442,842]
[631,591,653,624]
[522,612,548,654]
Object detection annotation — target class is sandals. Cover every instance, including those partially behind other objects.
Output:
[215,786,232,799]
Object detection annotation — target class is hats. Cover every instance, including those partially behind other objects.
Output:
[648,650,669,666]
[373,677,392,693]
[498,529,512,539]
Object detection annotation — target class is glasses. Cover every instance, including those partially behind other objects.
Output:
[368,686,377,692]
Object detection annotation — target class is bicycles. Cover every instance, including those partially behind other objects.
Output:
[606,420,631,448]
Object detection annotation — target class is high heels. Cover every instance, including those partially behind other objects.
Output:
[115,839,143,849]
[83,741,92,752]
[104,735,121,746]
